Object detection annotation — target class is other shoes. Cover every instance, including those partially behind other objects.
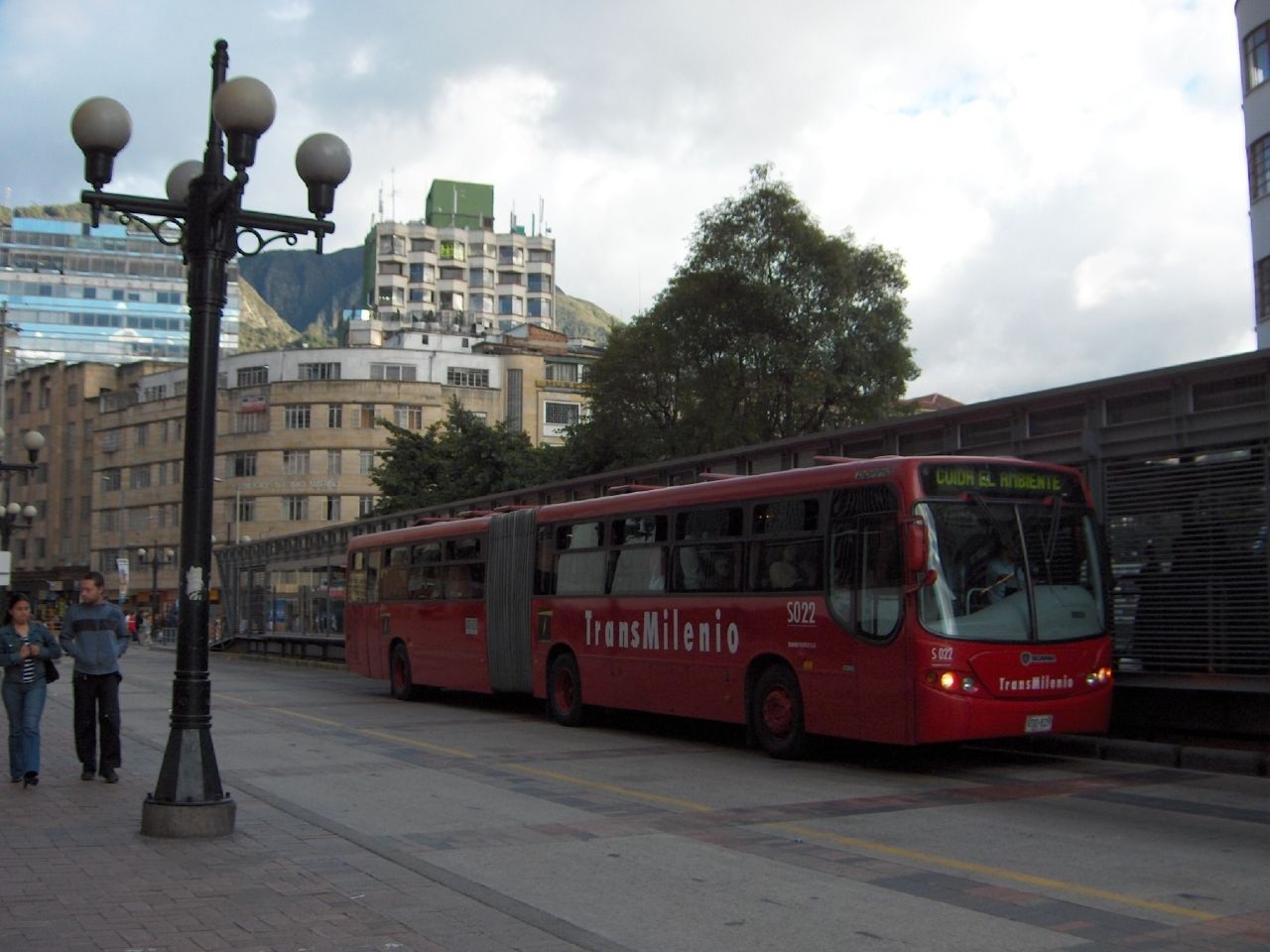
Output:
[80,762,97,781]
[98,765,119,783]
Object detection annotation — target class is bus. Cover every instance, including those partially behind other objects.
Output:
[341,449,1120,769]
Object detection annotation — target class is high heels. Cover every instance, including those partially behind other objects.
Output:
[24,773,39,788]
[11,775,22,782]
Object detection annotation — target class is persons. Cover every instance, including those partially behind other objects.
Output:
[0,592,62,785]
[667,519,902,591]
[59,571,129,784]
[123,609,180,648]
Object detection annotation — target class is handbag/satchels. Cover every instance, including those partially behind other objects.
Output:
[42,636,60,683]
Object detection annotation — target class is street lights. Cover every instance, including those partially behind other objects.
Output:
[0,423,46,631]
[136,539,175,636]
[66,38,353,841]
